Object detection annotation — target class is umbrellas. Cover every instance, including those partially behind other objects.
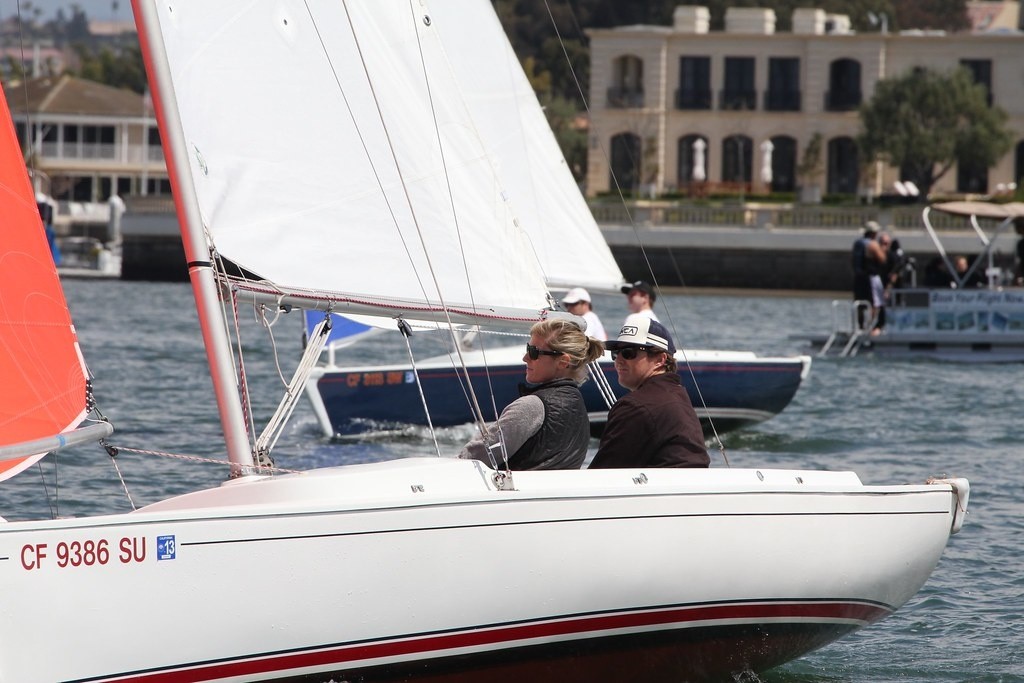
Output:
[694,137,707,183]
[760,140,774,184]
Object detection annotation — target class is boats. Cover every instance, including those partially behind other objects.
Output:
[786,198,1023,363]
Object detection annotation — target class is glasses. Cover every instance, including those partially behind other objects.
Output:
[526,342,564,360]
[611,344,648,360]
[564,302,581,308]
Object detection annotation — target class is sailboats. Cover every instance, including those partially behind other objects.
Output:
[0,1,971,683]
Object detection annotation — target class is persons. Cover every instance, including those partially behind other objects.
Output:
[620,281,659,324]
[562,288,607,342]
[586,316,711,468]
[457,318,603,471]
[851,219,988,337]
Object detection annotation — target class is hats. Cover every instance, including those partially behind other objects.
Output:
[621,281,657,299]
[561,288,591,304]
[866,221,880,232]
[600,317,677,356]
